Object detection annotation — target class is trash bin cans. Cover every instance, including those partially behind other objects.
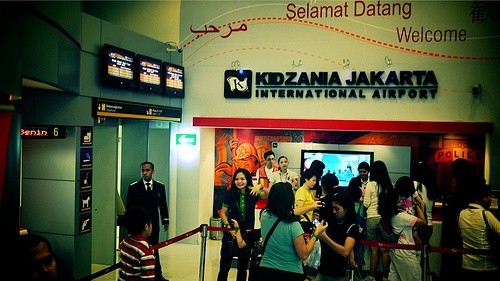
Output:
[211,217,223,239]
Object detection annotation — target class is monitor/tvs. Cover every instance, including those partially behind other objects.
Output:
[99,43,185,98]
[300,150,375,195]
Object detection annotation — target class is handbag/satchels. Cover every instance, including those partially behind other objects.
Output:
[416,214,434,245]
[375,221,403,244]
[301,213,314,235]
[482,209,500,268]
[250,244,264,269]
[355,202,366,240]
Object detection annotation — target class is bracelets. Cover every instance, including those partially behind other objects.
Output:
[234,227,240,232]
[312,234,319,241]
[223,223,230,227]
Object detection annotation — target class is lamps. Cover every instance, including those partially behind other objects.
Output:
[342,57,350,70]
[292,59,303,68]
[385,56,392,68]
[230,60,241,71]
[167,41,184,55]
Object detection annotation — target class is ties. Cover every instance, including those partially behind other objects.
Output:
[145,183,152,199]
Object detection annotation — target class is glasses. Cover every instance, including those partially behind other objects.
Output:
[266,158,274,161]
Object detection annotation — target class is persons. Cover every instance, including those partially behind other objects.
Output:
[117,204,158,281]
[120,161,170,281]
[249,150,433,281]
[11,233,58,281]
[217,168,270,281]
[440,158,499,281]
[116,191,126,226]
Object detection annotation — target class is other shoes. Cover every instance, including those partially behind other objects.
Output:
[154,273,169,281]
[383,278,388,281]
[362,276,375,281]
